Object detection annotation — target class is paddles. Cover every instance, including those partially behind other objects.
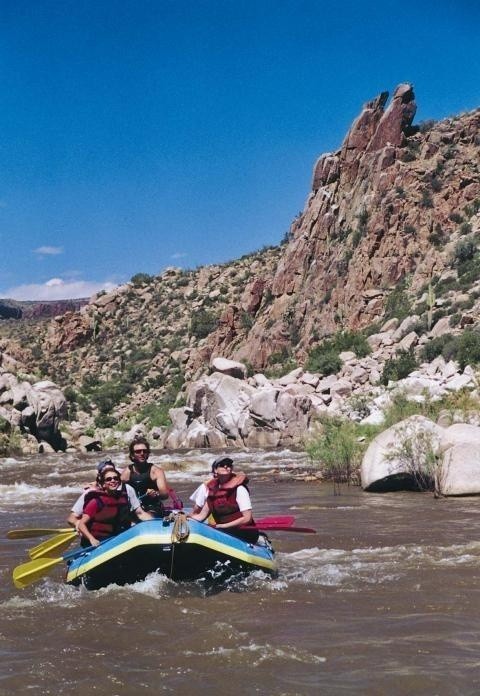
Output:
[13,536,117,590]
[209,523,317,533]
[28,493,149,560]
[254,515,295,529]
[7,527,79,540]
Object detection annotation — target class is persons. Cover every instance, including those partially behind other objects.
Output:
[187,477,216,525]
[120,437,170,516]
[78,469,143,546]
[67,460,153,532]
[187,455,260,545]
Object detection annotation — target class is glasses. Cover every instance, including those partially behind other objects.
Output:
[133,449,149,455]
[104,476,120,482]
[217,463,232,468]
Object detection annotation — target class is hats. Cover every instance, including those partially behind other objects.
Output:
[212,456,233,471]
[96,460,114,483]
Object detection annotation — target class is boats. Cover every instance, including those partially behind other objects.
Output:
[66,508,279,591]
